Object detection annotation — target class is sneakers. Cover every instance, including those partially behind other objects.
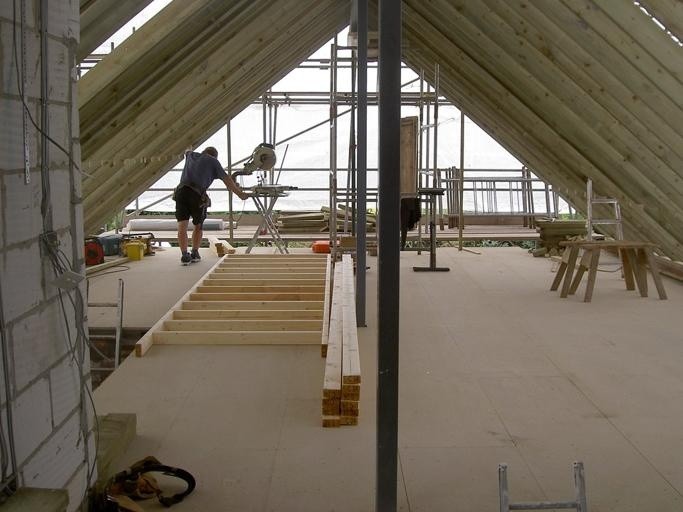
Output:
[180,252,191,266]
[190,250,202,262]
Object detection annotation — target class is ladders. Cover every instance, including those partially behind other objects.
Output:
[499,462,585,512]
[83,279,124,374]
[587,178,626,278]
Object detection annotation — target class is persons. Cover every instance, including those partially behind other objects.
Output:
[171,142,248,264]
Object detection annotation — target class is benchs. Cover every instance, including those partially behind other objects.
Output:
[568,243,668,302]
[549,240,648,298]
